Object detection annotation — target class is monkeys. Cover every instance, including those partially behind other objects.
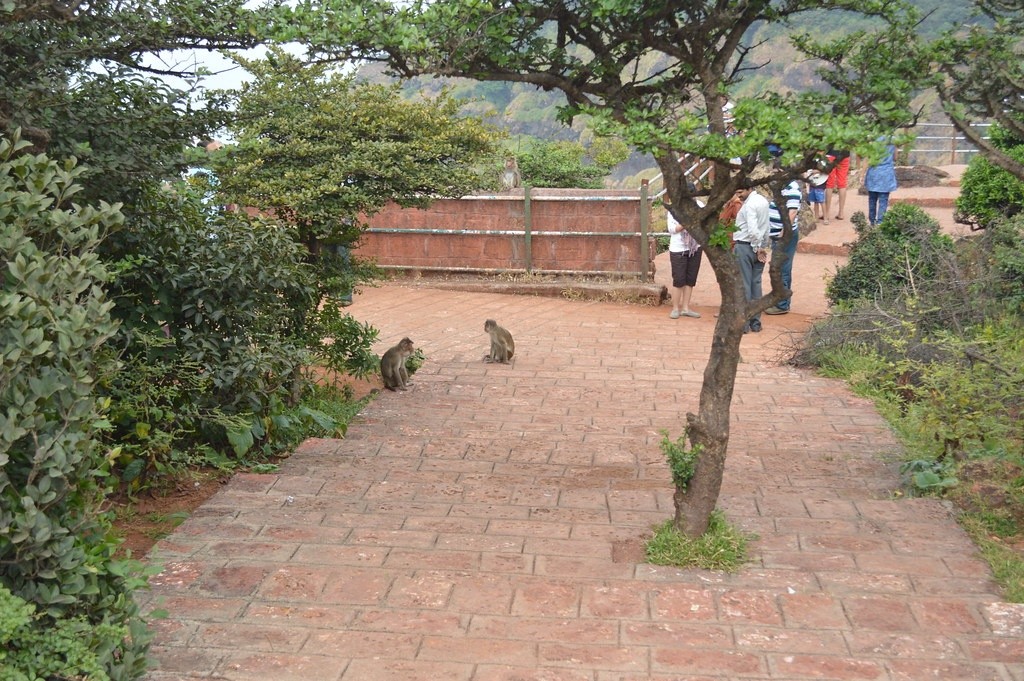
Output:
[481,318,515,365]
[380,336,415,392]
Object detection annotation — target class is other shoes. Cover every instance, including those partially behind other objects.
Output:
[670,309,680,318]
[681,309,700,318]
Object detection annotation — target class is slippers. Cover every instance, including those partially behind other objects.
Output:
[818,215,823,220]
[836,216,844,220]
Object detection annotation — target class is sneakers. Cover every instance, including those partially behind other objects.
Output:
[764,306,790,314]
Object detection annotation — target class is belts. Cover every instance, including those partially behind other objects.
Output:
[736,240,749,244]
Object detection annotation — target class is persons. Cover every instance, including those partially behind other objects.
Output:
[726,125,745,165]
[713,196,743,318]
[819,143,850,220]
[185,140,237,239]
[766,144,784,156]
[733,177,770,333]
[860,129,898,227]
[802,150,829,224]
[763,162,803,315]
[667,180,705,319]
